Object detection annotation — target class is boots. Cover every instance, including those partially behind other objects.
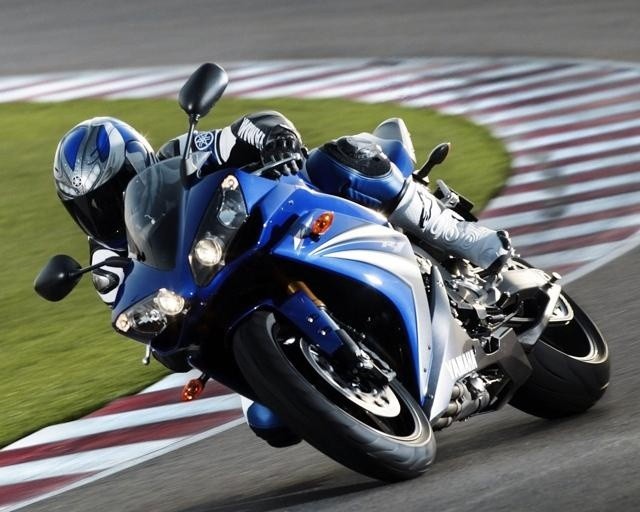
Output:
[389,177,511,275]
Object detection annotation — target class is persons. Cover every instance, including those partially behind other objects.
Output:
[51,115,514,450]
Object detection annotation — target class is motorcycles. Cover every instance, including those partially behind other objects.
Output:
[34,62,612,483]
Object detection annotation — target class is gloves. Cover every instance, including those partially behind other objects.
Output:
[261,125,303,178]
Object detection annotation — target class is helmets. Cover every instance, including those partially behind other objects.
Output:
[54,117,162,252]
